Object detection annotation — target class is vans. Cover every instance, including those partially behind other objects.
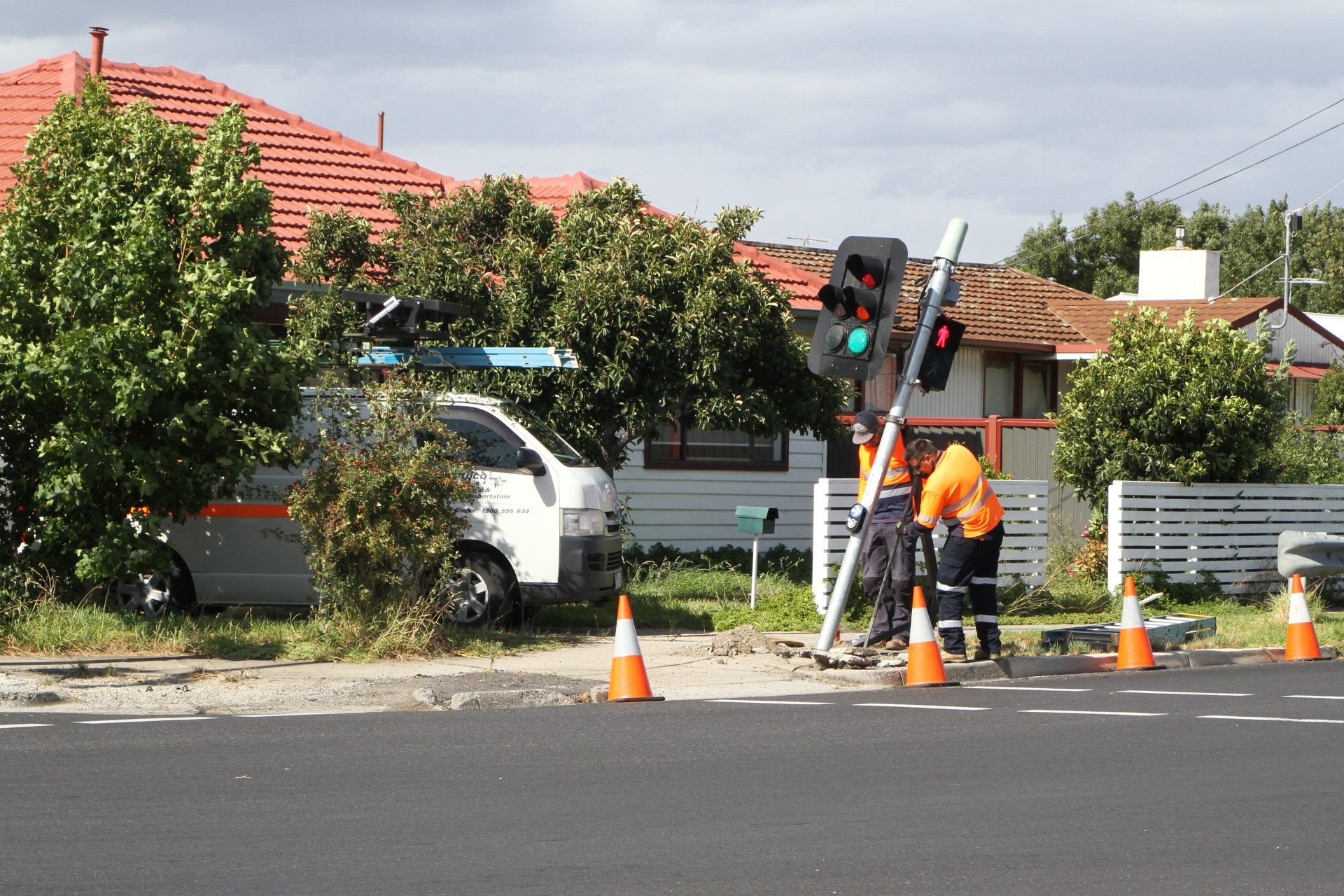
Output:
[16,388,627,634]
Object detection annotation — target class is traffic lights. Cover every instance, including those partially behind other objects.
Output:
[806,235,908,381]
[918,313,967,391]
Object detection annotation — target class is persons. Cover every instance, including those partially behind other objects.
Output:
[853,410,919,648]
[896,439,1005,663]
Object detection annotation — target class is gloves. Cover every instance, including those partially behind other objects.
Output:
[895,521,908,534]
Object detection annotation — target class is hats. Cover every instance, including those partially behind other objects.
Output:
[853,411,879,444]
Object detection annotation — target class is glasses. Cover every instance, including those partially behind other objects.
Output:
[914,453,931,469]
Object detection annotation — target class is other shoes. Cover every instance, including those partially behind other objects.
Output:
[886,639,906,650]
[940,649,968,663]
[975,647,1001,660]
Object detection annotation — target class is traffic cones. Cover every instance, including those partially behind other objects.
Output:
[1283,573,1333,661]
[1108,576,1167,672]
[608,594,674,702]
[901,585,960,688]
